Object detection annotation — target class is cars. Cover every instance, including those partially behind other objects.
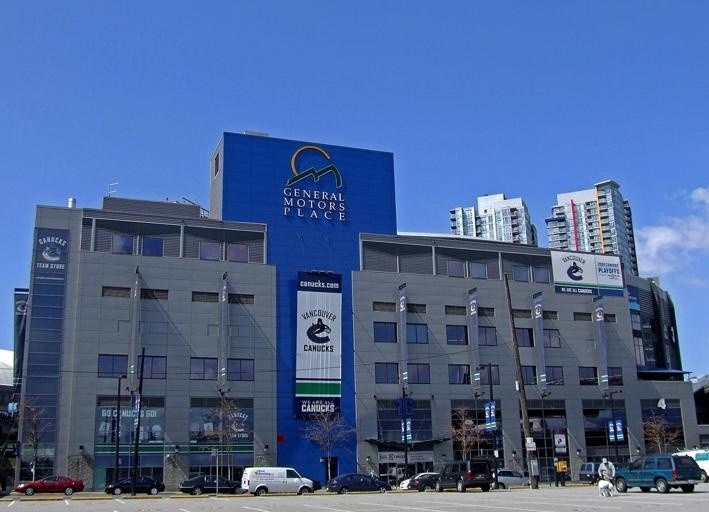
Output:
[15,476,84,496]
[179,475,241,496]
[399,459,529,493]
[105,476,165,496]
[326,473,391,495]
[615,455,701,494]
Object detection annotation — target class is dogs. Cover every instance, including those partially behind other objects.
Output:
[597,479,619,497]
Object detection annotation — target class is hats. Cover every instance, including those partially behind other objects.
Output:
[603,458,608,464]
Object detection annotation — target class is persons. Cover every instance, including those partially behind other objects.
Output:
[597,457,616,496]
[596,477,613,497]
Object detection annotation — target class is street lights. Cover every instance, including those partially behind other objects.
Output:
[114,375,127,482]
[476,362,499,489]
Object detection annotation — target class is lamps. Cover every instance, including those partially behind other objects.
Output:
[175,445,180,453]
[79,445,84,453]
[366,456,371,462]
[442,454,447,459]
[636,447,641,452]
[167,454,171,459]
[512,451,517,457]
[320,456,325,463]
[577,449,582,456]
[264,445,269,452]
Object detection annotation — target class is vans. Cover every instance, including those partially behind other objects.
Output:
[671,448,709,483]
[580,462,623,486]
[241,467,314,496]
